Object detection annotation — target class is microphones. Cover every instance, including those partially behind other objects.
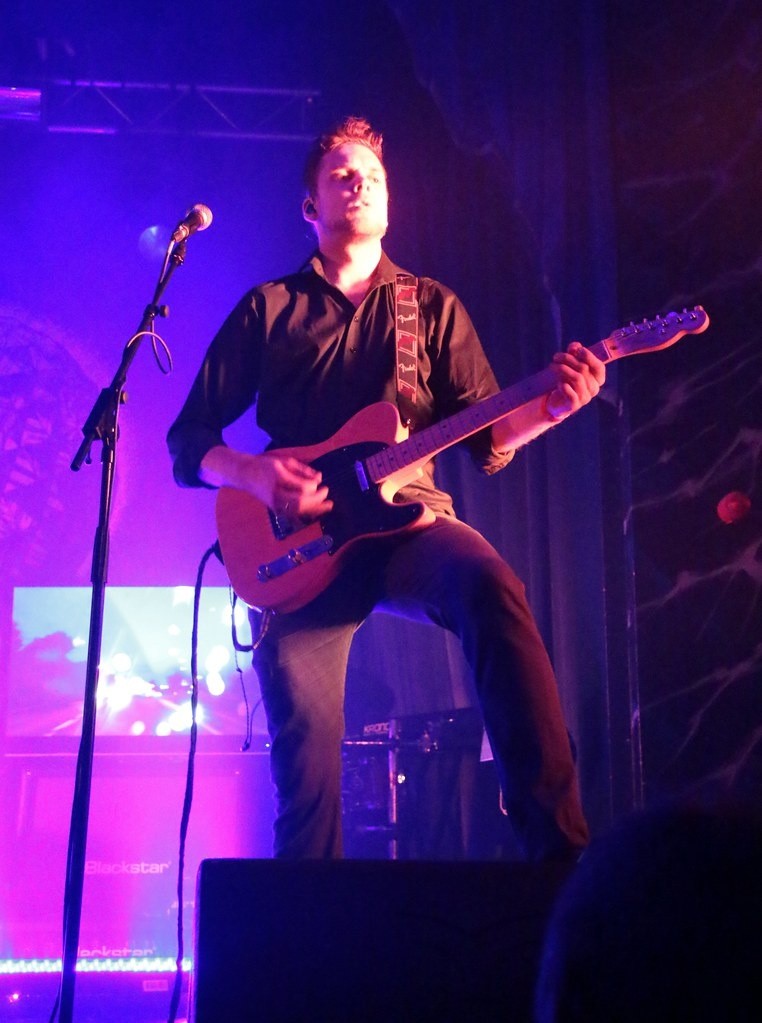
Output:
[172,205,213,242]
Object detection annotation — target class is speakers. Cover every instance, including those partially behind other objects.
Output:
[192,856,573,1023]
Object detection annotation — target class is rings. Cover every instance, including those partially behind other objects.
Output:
[284,502,289,512]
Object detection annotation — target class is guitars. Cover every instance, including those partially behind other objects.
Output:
[213,302,713,620]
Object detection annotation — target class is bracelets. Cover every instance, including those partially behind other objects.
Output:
[541,391,560,423]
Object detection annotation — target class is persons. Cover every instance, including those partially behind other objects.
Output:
[164,114,608,867]
